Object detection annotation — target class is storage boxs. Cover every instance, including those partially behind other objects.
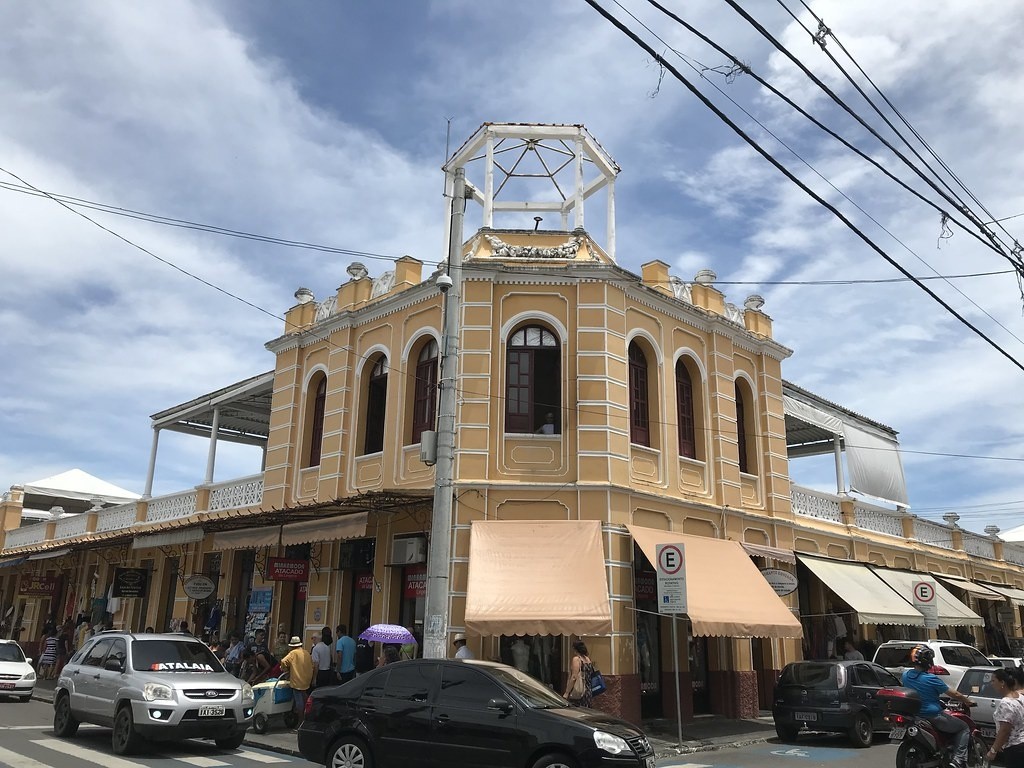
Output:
[251,678,293,716]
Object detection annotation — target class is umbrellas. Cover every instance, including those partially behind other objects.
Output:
[358,624,419,644]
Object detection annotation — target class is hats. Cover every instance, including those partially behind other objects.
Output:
[452,634,467,643]
[287,636,303,646]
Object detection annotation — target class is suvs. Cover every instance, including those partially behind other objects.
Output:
[870,638,995,698]
[53,629,257,756]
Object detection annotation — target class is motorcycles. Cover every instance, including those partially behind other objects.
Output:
[875,684,991,768]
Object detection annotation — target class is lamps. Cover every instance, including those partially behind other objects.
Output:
[534,217,543,230]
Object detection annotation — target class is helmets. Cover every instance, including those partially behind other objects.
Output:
[912,645,934,668]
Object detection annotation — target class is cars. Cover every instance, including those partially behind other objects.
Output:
[297,657,656,768]
[772,659,904,748]
[0,639,37,704]
[954,657,1024,743]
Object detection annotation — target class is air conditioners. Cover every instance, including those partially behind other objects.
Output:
[390,538,426,564]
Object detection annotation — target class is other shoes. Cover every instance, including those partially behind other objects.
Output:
[37,674,43,679]
[46,676,53,680]
[949,759,966,768]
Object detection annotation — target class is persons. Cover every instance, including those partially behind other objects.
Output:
[280,636,313,733]
[510,639,530,673]
[689,638,699,683]
[399,627,418,660]
[453,633,475,660]
[902,644,1024,768]
[310,626,340,692]
[336,624,357,683]
[376,642,402,670]
[960,630,972,645]
[201,629,293,686]
[146,627,154,633]
[178,621,192,635]
[32,612,113,680]
[0,606,25,640]
[536,412,554,433]
[563,639,595,708]
[637,630,651,688]
[830,637,864,660]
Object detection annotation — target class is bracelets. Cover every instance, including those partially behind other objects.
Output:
[990,747,997,754]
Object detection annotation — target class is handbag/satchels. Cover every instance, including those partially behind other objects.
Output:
[590,667,607,697]
[566,657,584,700]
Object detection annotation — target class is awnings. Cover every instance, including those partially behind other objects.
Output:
[464,520,612,637]
[929,572,1024,606]
[625,523,804,639]
[868,564,986,628]
[0,487,435,558]
[9,467,143,522]
[795,552,925,626]
